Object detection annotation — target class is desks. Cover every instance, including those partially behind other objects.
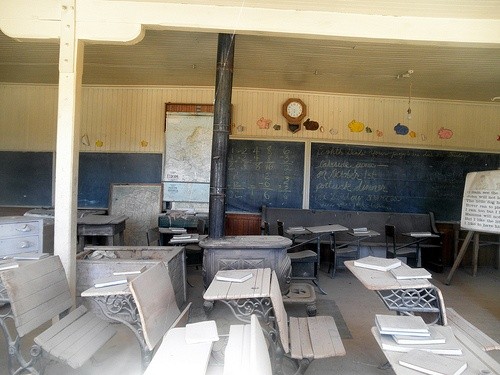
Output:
[436,220,464,262]
[401,232,440,268]
[77,215,129,254]
[370,326,496,375]
[81,274,145,348]
[346,259,443,325]
[305,224,381,277]
[203,268,270,329]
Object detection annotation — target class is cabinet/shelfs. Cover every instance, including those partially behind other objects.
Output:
[0,216,43,306]
[225,211,263,236]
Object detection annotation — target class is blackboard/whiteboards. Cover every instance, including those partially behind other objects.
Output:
[78,152,166,212]
[307,139,500,225]
[225,138,308,214]
[0,151,55,208]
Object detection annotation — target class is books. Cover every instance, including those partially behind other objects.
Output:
[169,227,187,232]
[95,274,126,287]
[14,252,49,260]
[351,227,371,233]
[410,232,431,236]
[216,270,253,283]
[184,319,218,343]
[389,268,432,279]
[112,265,146,274]
[354,256,401,272]
[0,260,19,269]
[399,348,467,375]
[374,315,463,355]
[170,233,199,242]
[287,227,305,232]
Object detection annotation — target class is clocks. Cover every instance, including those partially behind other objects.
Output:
[282,98,307,134]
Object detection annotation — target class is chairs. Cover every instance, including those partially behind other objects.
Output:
[146,218,206,270]
[264,219,318,278]
[385,224,421,268]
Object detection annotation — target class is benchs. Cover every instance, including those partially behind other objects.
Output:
[262,204,447,277]
[0,256,500,375]
